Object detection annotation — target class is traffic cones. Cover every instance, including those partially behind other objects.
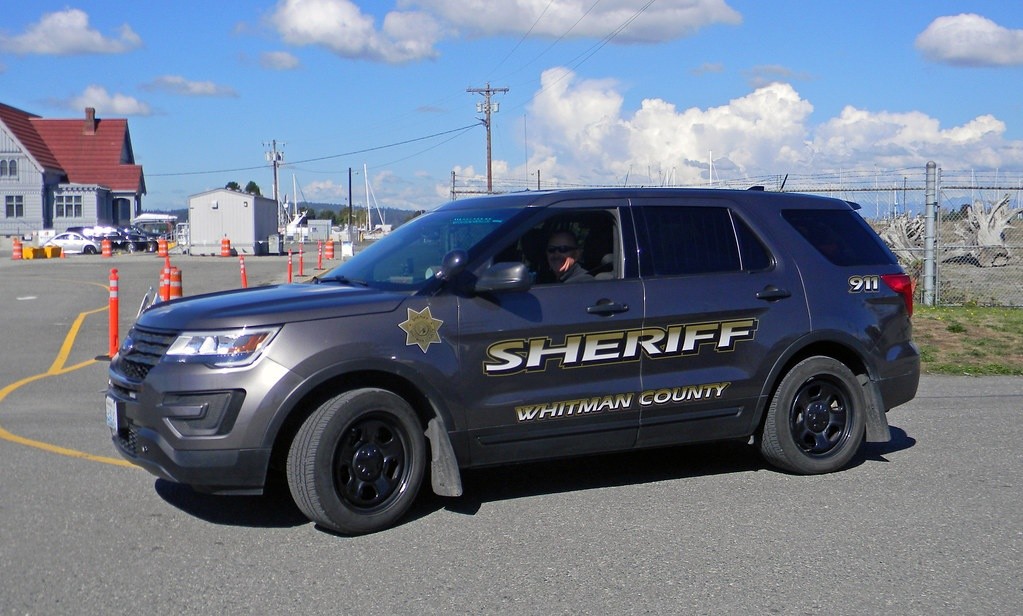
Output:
[58,245,65,259]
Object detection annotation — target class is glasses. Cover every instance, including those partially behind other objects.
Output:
[545,244,581,253]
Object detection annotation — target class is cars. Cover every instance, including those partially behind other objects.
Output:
[36,223,172,255]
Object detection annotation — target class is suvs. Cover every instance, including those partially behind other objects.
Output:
[103,186,923,540]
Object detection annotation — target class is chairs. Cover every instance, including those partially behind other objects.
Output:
[583,224,613,278]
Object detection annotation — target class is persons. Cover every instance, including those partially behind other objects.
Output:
[543,232,595,284]
[516,228,540,271]
